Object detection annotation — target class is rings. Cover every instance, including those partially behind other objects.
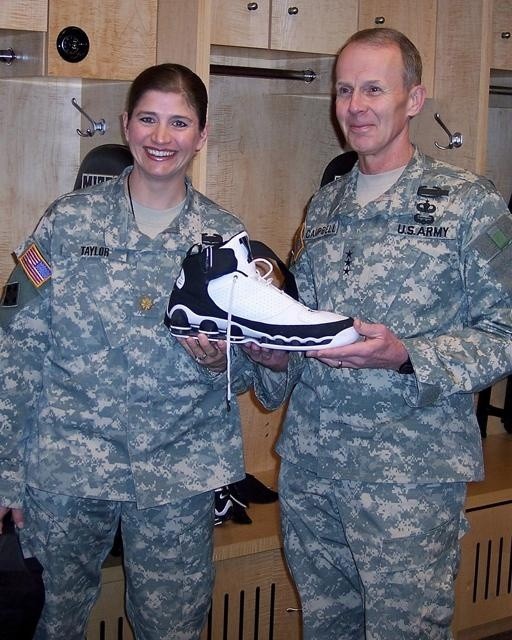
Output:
[198,355,209,361]
[339,360,344,369]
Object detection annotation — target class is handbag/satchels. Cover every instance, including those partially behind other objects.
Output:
[0,509,46,640]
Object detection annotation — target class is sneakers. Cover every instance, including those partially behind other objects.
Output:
[215,487,246,525]
[162,230,361,351]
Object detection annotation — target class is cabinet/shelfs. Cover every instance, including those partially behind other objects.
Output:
[359,0,439,104]
[453,502,512,640]
[0,0,52,37]
[0,1,162,86]
[210,0,361,61]
[85,547,304,639]
[490,0,511,73]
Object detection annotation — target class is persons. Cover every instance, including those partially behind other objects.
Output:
[241,27,512,639]
[0,62,255,640]
